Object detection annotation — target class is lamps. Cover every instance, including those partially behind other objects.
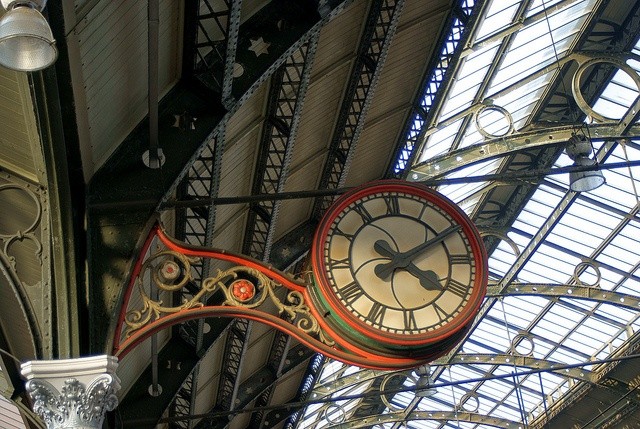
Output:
[540,1,607,193]
[2,1,60,74]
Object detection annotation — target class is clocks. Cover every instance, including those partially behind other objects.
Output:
[305,178,489,359]
